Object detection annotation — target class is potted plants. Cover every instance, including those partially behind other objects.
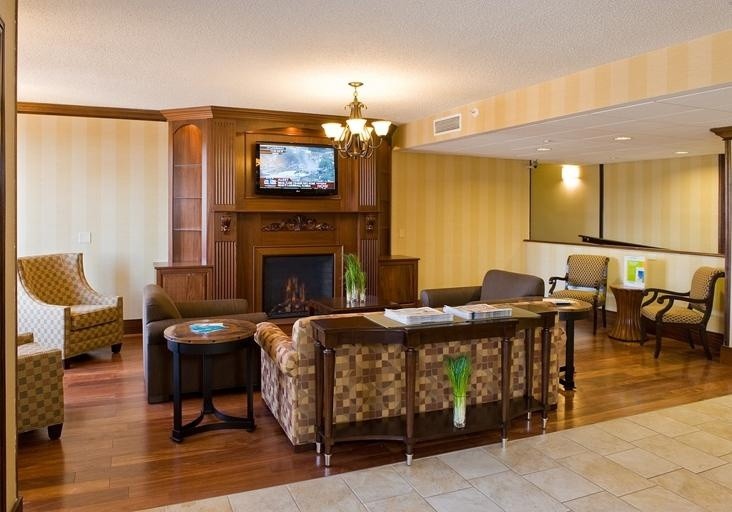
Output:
[343,252,367,301]
[445,351,475,430]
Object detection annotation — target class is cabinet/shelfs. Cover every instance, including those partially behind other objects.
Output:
[151,262,215,305]
[378,253,421,303]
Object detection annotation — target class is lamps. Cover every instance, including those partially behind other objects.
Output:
[321,82,395,161]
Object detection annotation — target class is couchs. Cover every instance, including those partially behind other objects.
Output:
[252,297,568,454]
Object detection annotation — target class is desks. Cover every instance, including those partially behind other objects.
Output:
[606,284,649,343]
[544,296,593,393]
[163,317,259,444]
[308,300,559,468]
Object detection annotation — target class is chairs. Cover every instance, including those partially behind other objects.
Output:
[138,282,269,404]
[15,331,66,441]
[16,251,126,367]
[421,269,545,307]
[638,265,726,362]
[548,254,610,338]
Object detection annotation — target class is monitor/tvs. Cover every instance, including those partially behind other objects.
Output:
[254,140,339,197]
